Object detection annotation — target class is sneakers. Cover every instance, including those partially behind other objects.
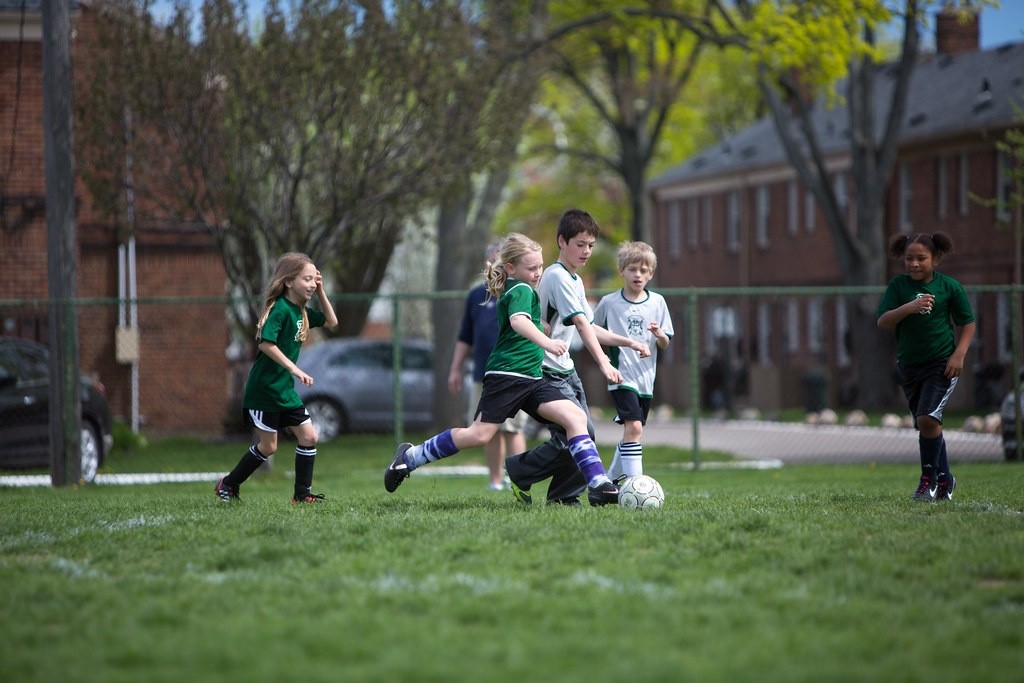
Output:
[384,442,413,493]
[912,475,939,501]
[215,477,242,504]
[546,498,581,507]
[291,492,328,504]
[504,458,533,505]
[935,472,956,501]
[587,473,627,507]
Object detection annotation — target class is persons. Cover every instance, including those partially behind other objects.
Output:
[384,231,627,507]
[593,240,675,484]
[874,232,977,503]
[502,206,652,505]
[215,251,339,505]
[447,235,527,490]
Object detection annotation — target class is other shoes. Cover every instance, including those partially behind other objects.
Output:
[491,467,513,491]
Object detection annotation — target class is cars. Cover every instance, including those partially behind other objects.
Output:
[282,338,439,443]
[1000,372,1024,462]
[0,337,115,490]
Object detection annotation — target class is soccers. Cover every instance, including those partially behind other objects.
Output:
[617,475,665,513]
